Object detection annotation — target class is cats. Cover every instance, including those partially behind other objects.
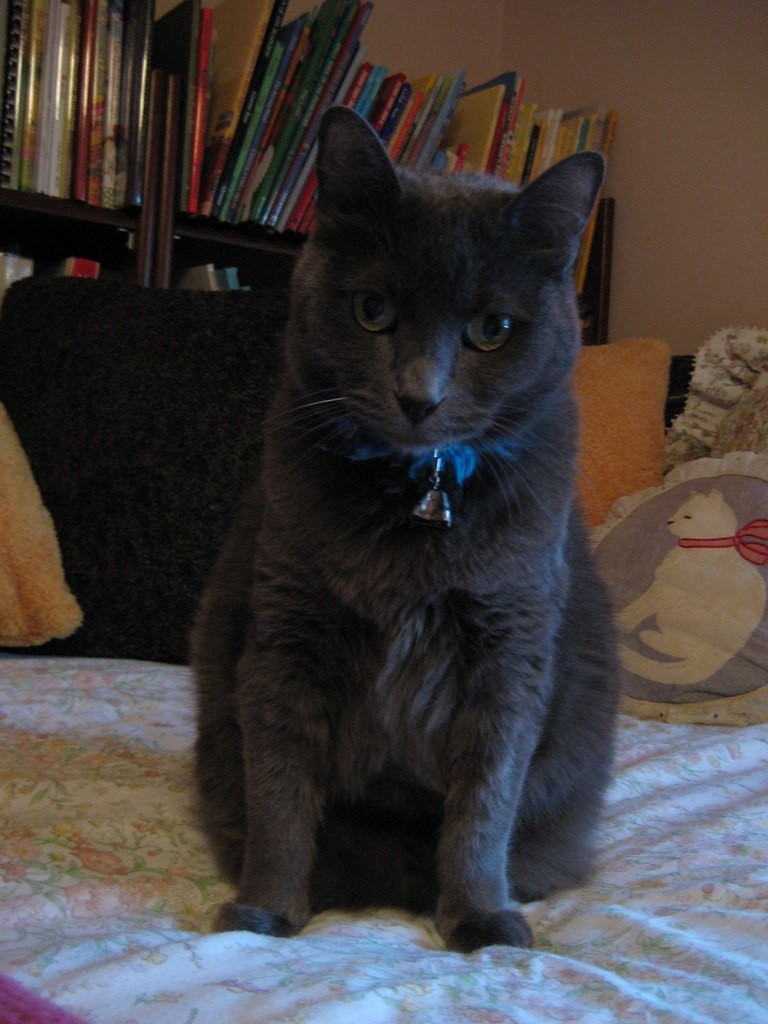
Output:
[181,108,620,957]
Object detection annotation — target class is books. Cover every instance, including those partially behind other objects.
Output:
[1,3,617,309]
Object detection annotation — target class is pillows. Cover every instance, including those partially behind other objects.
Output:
[579,452,768,729]
[0,272,281,666]
[539,333,673,540]
